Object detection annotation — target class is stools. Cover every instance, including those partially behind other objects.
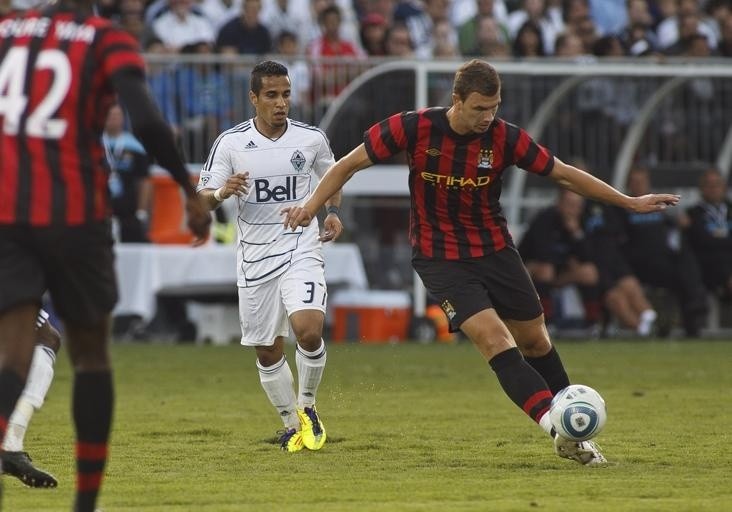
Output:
[329,288,410,345]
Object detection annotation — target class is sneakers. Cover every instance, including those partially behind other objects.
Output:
[554,434,607,466]
[1,449,57,487]
[276,403,326,451]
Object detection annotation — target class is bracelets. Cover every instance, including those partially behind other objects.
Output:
[212,184,227,203]
[326,203,342,213]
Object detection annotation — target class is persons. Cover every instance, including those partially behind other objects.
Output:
[0,308,63,490]
[0,1,731,342]
[197,58,346,456]
[0,0,213,512]
[277,58,683,468]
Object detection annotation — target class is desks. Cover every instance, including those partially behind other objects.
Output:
[110,238,372,340]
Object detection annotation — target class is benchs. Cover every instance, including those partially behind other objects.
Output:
[142,279,355,343]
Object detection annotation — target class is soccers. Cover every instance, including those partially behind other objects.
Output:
[550,384,607,442]
